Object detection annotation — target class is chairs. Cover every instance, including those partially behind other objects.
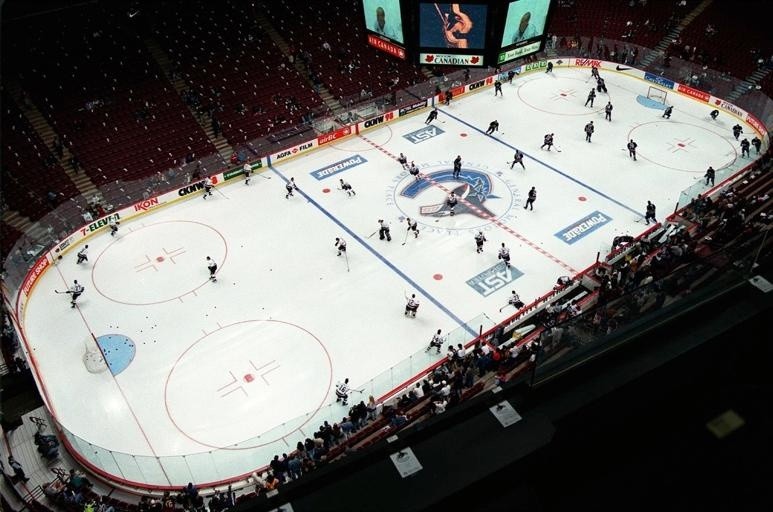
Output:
[646,61,772,140]
[26,477,289,512]
[320,352,528,465]
[0,1,221,274]
[660,160,772,290]
[545,1,773,61]
[223,4,462,165]
